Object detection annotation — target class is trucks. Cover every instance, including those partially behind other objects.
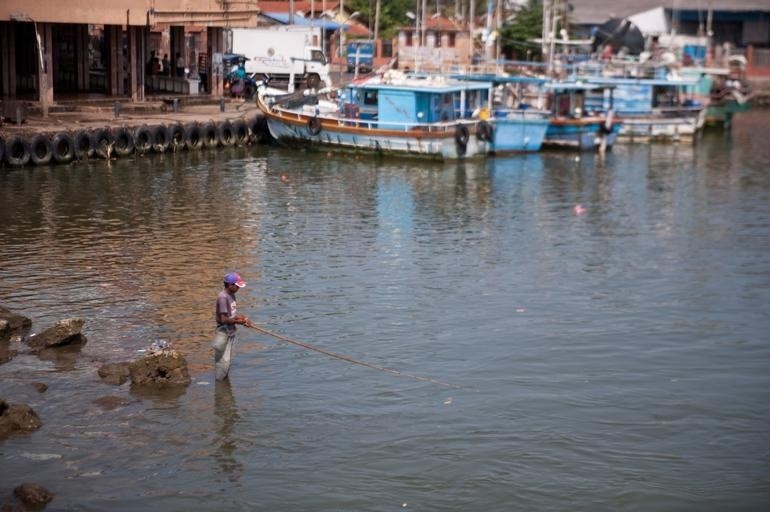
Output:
[232,26,332,89]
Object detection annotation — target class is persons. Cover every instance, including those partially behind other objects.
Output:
[214,272,250,380]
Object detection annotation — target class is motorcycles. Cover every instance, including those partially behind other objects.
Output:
[196,50,257,98]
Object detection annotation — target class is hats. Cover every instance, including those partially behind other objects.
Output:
[224,273,245,287]
